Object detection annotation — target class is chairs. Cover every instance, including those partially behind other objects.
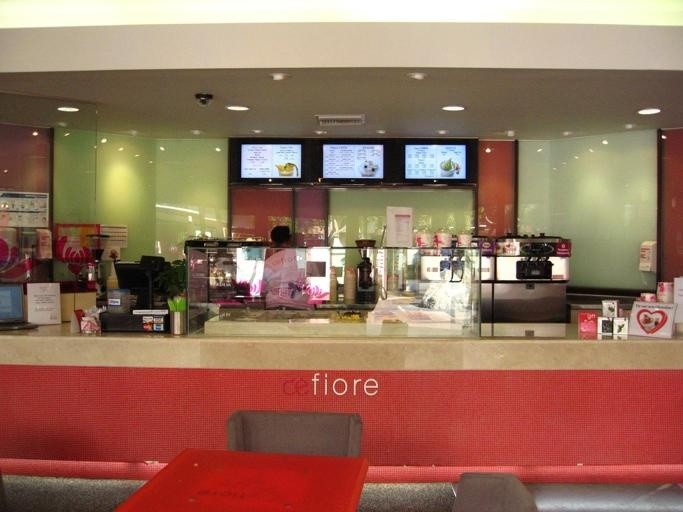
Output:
[450,473,537,512]
[226,409,364,457]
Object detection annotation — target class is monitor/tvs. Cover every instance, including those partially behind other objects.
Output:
[403,140,469,187]
[239,142,304,185]
[321,142,386,186]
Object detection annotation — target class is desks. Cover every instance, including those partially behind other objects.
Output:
[117,447,368,512]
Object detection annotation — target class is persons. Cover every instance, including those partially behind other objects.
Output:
[264,225,322,309]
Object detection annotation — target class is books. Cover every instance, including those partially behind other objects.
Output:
[576,299,629,336]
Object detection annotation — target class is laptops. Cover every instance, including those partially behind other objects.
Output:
[0,282,39,330]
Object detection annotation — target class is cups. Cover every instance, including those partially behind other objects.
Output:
[170,311,186,335]
[344,267,357,303]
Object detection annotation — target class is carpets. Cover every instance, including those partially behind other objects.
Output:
[2,475,456,512]
